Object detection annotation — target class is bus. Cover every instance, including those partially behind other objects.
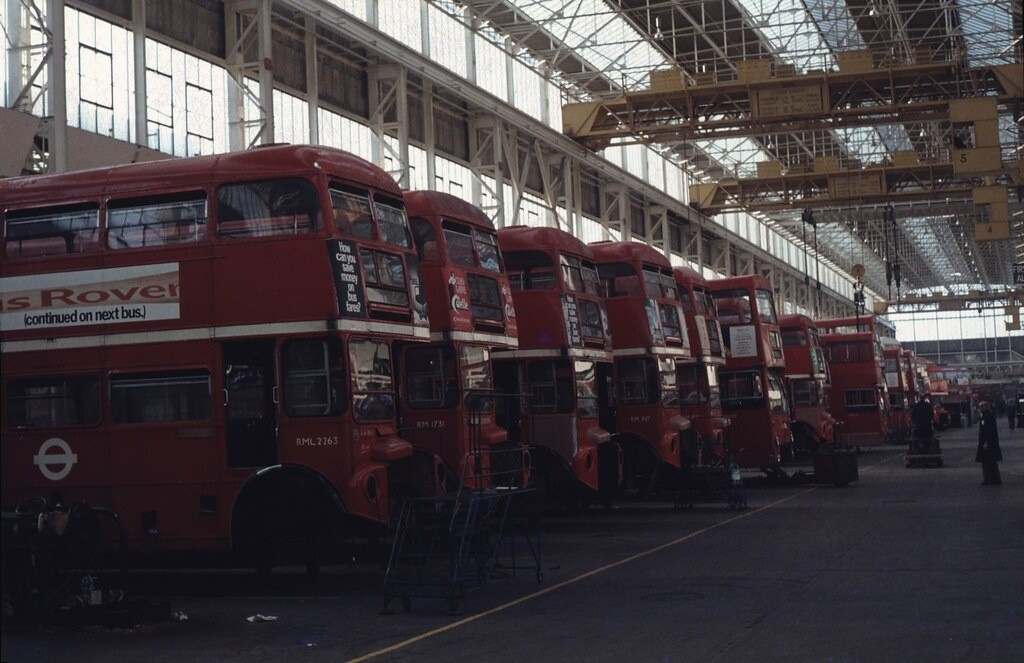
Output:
[502,239,702,511]
[582,264,983,499]
[2,144,432,595]
[416,224,616,515]
[0,190,534,561]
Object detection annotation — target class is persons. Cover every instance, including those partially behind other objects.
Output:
[911,394,934,454]
[1006,400,1016,430]
[975,401,1003,485]
